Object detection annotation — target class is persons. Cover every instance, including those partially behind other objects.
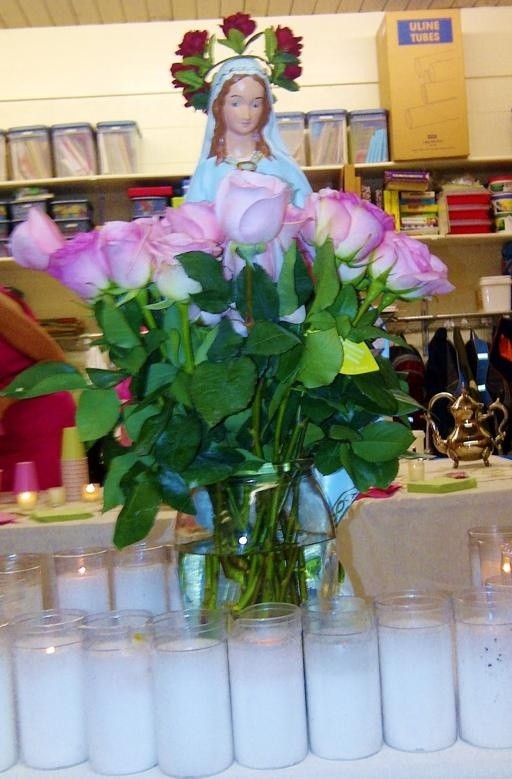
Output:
[183,57,389,599]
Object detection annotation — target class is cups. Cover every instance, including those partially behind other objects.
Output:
[149,608,235,775]
[0,550,46,642]
[453,586,510,749]
[403,428,425,480]
[51,546,111,642]
[9,462,39,494]
[300,594,383,762]
[58,426,90,504]
[0,618,18,771]
[114,543,171,620]
[47,485,65,507]
[225,600,308,771]
[372,590,460,755]
[76,608,158,774]
[12,607,87,771]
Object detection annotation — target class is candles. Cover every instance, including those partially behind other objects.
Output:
[49,487,66,506]
[16,491,37,509]
[82,484,100,501]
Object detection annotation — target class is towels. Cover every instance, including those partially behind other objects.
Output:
[354,483,402,501]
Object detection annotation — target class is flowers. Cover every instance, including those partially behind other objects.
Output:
[7,172,456,615]
[170,13,293,115]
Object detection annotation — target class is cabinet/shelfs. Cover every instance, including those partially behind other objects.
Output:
[0,156,512,262]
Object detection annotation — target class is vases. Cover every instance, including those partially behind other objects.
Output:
[172,468,340,640]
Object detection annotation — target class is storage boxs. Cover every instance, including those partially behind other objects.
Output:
[479,274,511,312]
[0,120,189,258]
[268,107,390,166]
[377,7,471,160]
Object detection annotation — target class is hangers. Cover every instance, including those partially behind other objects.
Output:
[390,311,512,348]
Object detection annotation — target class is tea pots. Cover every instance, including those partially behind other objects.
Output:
[422,385,509,469]
